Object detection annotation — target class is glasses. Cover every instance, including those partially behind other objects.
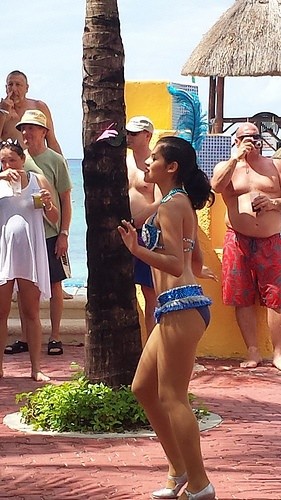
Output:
[0,137,21,148]
[126,130,146,136]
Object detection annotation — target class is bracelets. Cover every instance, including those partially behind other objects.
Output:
[235,158,240,162]
[45,202,52,211]
[0,109,9,115]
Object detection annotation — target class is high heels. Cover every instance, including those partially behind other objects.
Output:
[152,469,188,500]
[177,482,215,500]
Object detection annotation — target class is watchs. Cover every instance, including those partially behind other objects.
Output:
[61,230,69,236]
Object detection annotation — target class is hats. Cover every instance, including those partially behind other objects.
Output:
[125,116,154,134]
[236,122,260,137]
[15,110,51,132]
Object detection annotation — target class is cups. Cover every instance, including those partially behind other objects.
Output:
[32,193,44,209]
[11,175,21,194]
[249,192,261,212]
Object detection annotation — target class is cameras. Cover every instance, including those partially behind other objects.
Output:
[250,139,263,149]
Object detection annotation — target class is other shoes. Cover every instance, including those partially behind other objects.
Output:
[32,370,50,381]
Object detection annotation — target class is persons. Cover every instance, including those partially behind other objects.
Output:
[211,122,281,369]
[119,136,219,500]
[124,116,163,348]
[0,138,60,380]
[3,109,72,355]
[0,70,74,301]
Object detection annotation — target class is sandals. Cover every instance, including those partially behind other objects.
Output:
[48,340,63,355]
[4,340,29,354]
